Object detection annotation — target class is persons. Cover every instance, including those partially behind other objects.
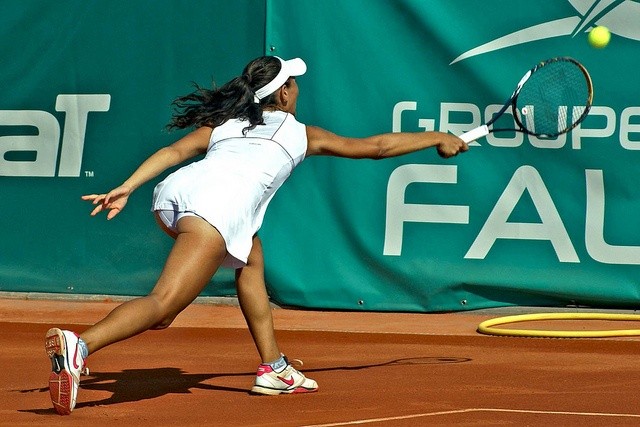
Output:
[45,56,469,415]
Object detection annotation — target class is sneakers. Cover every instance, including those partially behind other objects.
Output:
[44,327,89,416]
[251,353,320,396]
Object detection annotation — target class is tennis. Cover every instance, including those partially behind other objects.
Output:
[588,26,610,48]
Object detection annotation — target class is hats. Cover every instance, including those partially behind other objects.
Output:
[254,55,307,105]
